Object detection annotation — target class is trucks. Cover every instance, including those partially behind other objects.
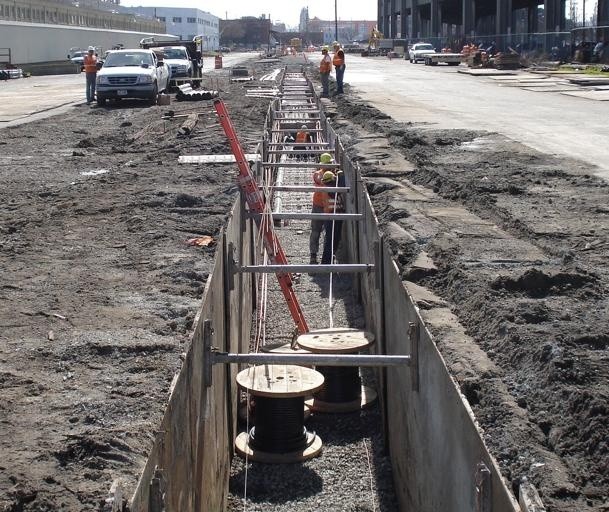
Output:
[141,35,205,88]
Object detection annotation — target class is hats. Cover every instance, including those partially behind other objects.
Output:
[88,45,95,53]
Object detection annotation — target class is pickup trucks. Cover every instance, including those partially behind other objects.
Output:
[95,49,173,105]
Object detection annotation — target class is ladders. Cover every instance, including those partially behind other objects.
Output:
[213,98,309,336]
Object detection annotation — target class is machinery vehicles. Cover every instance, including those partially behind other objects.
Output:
[366,26,387,58]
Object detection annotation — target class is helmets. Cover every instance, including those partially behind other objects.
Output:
[318,152,336,165]
[330,40,341,48]
[321,170,337,184]
[321,46,329,52]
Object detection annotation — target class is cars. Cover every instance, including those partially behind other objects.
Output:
[66,49,113,72]
[470,39,608,64]
[408,43,436,66]
[215,46,231,54]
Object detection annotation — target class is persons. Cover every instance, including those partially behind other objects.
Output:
[309,153,335,264]
[293,125,311,157]
[320,48,332,97]
[83,45,98,101]
[322,170,346,264]
[331,41,345,94]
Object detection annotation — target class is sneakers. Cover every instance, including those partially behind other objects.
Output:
[308,260,318,277]
[86,98,96,106]
